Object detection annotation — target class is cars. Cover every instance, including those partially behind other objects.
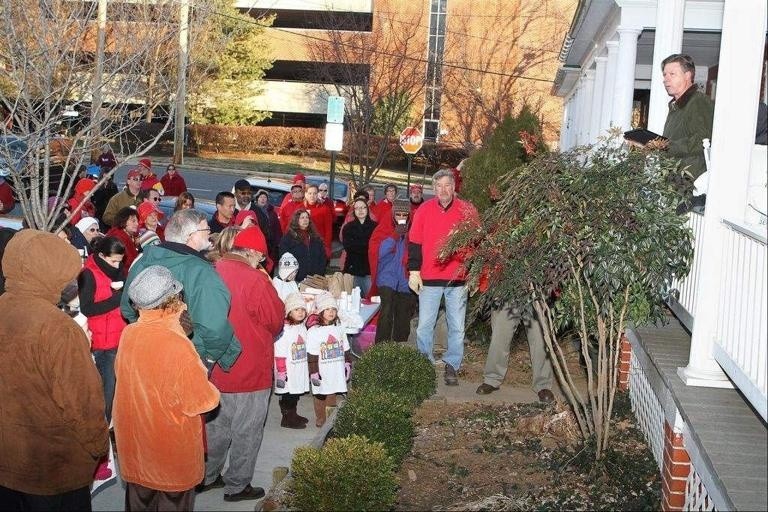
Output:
[155,194,219,226]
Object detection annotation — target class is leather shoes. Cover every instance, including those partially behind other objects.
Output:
[224,483,265,501]
[195,474,225,493]
[476,383,499,394]
[538,389,554,404]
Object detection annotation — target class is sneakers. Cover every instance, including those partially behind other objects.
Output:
[444,364,459,385]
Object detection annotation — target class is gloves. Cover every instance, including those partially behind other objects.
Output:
[395,224,408,235]
[310,372,321,386]
[408,271,423,295]
[276,372,288,388]
[345,362,351,381]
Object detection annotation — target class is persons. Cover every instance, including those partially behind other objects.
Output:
[1,176,14,215]
[50,144,423,481]
[195,227,285,501]
[410,170,555,404]
[646,53,714,216]
[1,229,222,511]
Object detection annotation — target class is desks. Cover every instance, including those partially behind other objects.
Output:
[345,302,379,360]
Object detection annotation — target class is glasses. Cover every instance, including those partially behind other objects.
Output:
[59,205,72,213]
[133,178,143,181]
[150,197,161,202]
[190,226,212,237]
[90,228,100,232]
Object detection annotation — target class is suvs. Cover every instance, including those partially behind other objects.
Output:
[230,164,352,227]
[0,135,82,203]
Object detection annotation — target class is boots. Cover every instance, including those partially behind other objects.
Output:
[279,399,308,429]
[94,462,112,480]
[314,394,336,427]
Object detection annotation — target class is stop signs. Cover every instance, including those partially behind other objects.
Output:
[398,124,424,156]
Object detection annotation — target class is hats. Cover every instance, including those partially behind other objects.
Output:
[137,202,164,224]
[384,182,422,195]
[235,180,251,191]
[48,196,69,215]
[279,252,299,282]
[75,178,95,193]
[294,173,305,184]
[233,224,267,256]
[139,228,161,250]
[128,159,151,178]
[75,217,99,234]
[284,290,306,317]
[315,290,338,314]
[127,265,183,310]
[392,200,411,226]
